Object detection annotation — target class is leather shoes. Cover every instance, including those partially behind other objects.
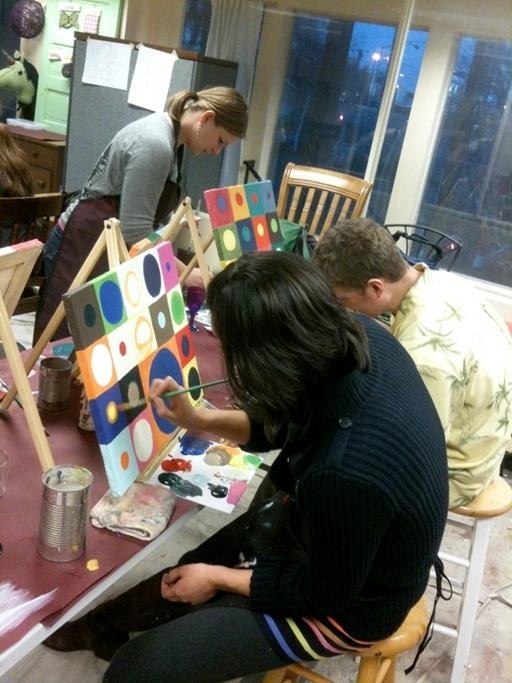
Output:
[42,614,129,663]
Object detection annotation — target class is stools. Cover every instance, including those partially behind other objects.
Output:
[429,474,512,683]
[261,587,426,683]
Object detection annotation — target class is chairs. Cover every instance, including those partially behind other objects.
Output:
[275,160,464,272]
[0,189,70,315]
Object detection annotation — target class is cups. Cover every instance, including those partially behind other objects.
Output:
[40,466,93,560]
[40,357,70,415]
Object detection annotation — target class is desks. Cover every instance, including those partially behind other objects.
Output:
[0,302,260,675]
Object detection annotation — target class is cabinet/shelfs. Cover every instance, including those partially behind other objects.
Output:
[1,122,66,193]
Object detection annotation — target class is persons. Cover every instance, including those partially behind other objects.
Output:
[42,250,451,682]
[0,121,41,247]
[32,83,251,348]
[309,216,512,516]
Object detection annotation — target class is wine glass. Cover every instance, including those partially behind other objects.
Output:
[184,285,206,332]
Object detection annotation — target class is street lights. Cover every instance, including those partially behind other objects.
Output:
[365,52,381,104]
